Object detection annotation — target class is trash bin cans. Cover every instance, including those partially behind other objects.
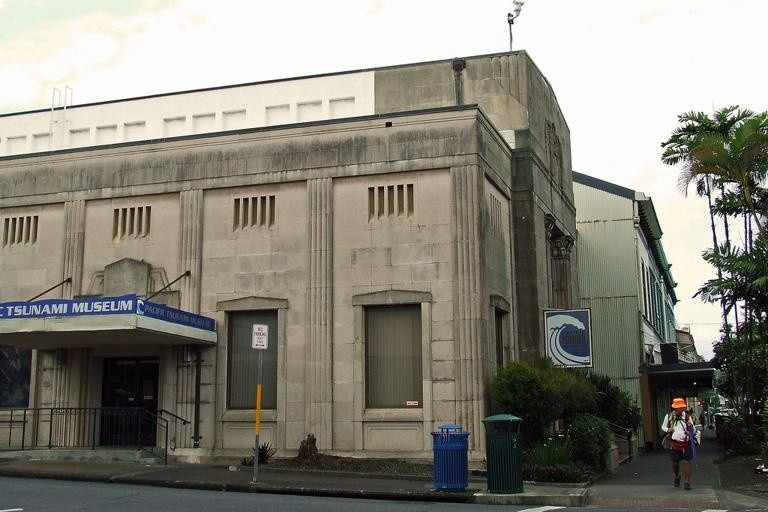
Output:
[430,423,470,490]
[482,414,523,493]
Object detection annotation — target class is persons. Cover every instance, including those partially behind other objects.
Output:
[661,398,696,490]
[699,411,706,431]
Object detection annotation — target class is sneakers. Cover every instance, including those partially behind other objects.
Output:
[684,482,690,490]
[674,475,681,487]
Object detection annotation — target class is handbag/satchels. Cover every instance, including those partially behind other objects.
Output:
[662,430,703,452]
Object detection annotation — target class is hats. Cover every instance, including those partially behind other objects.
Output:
[672,398,686,408]
